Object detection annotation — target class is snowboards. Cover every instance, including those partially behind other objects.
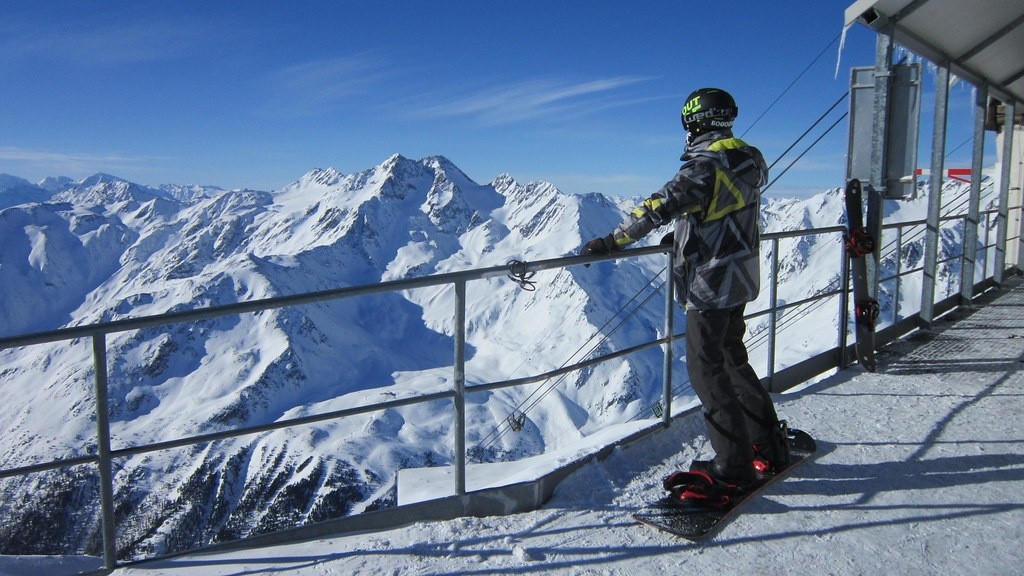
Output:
[631,427,817,542]
[844,178,881,373]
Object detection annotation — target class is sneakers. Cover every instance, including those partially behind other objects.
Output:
[689,455,755,488]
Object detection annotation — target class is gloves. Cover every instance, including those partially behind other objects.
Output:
[660,231,674,255]
[580,233,619,269]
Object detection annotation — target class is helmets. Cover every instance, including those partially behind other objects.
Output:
[680,88,739,131]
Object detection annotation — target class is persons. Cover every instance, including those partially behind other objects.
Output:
[580,87,790,486]
